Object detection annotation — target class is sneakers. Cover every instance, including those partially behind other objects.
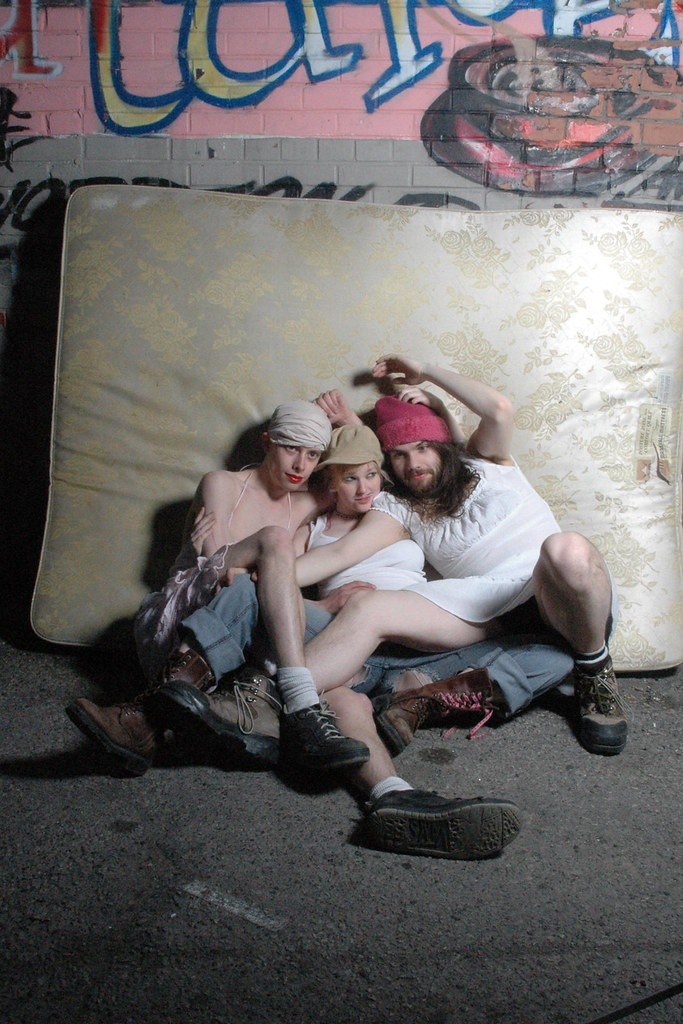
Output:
[572,656,635,757]
[154,673,283,771]
[362,790,523,859]
[279,704,370,774]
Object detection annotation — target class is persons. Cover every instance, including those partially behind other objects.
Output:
[159,350,627,765]
[131,389,520,859]
[66,424,573,768]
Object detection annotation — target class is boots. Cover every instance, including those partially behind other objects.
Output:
[65,647,216,778]
[372,663,498,754]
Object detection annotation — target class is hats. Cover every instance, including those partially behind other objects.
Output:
[374,394,453,453]
[313,424,385,474]
